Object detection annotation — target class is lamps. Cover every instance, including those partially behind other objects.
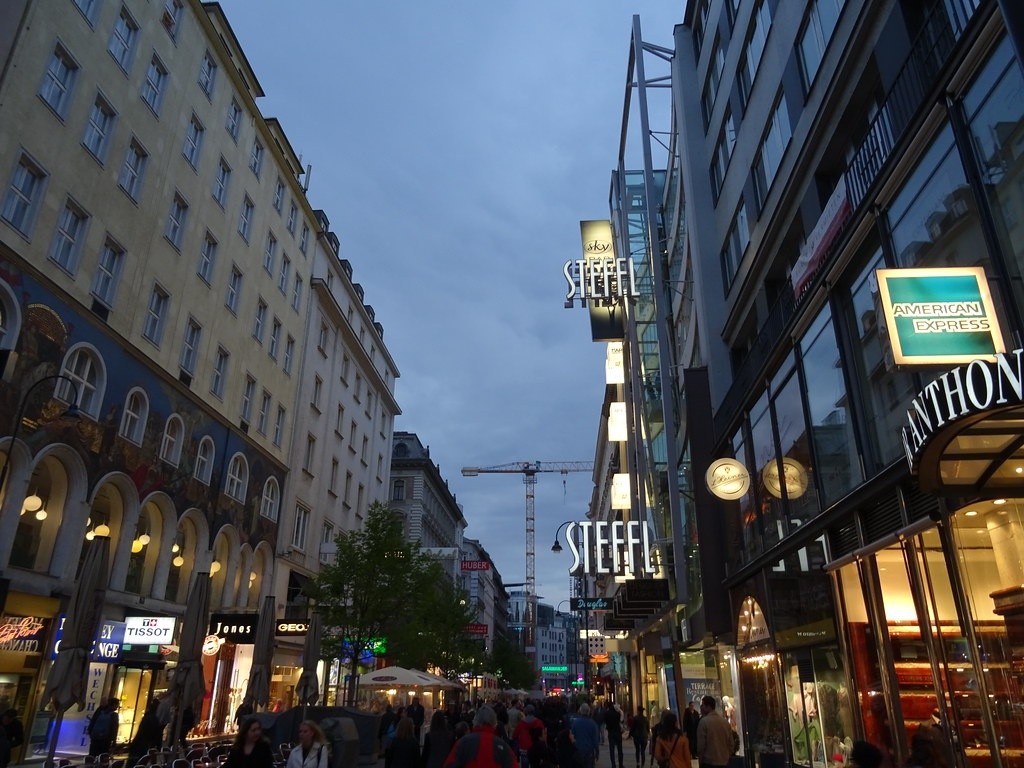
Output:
[173,534,184,566]
[140,596,146,604]
[281,545,293,556]
[20,485,48,521]
[131,527,151,553]
[249,569,256,587]
[210,552,220,578]
[86,517,110,541]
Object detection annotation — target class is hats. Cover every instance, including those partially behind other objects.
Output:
[580,703,590,715]
[524,705,536,716]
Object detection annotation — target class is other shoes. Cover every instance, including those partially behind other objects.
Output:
[637,765,640,768]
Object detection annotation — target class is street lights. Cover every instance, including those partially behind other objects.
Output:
[555,599,578,688]
[550,522,592,711]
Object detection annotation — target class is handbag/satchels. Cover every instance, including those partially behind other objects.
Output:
[659,758,670,768]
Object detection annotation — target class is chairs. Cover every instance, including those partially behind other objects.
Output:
[42,738,298,768]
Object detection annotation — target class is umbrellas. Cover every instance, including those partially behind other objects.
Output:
[157,573,215,763]
[41,536,111,768]
[295,613,321,718]
[243,596,275,715]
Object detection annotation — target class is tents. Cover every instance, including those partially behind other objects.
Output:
[346,665,462,721]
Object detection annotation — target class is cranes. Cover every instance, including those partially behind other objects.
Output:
[461,459,595,663]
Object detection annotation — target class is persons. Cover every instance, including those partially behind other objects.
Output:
[1,690,963,768]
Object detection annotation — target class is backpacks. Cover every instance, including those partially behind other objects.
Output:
[91,711,114,739]
